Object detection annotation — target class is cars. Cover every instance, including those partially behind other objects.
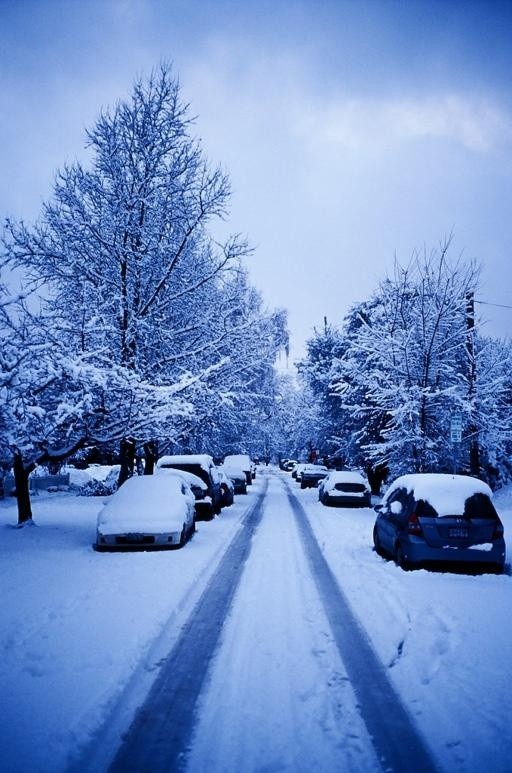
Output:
[97,455,256,551]
[280,459,505,573]
